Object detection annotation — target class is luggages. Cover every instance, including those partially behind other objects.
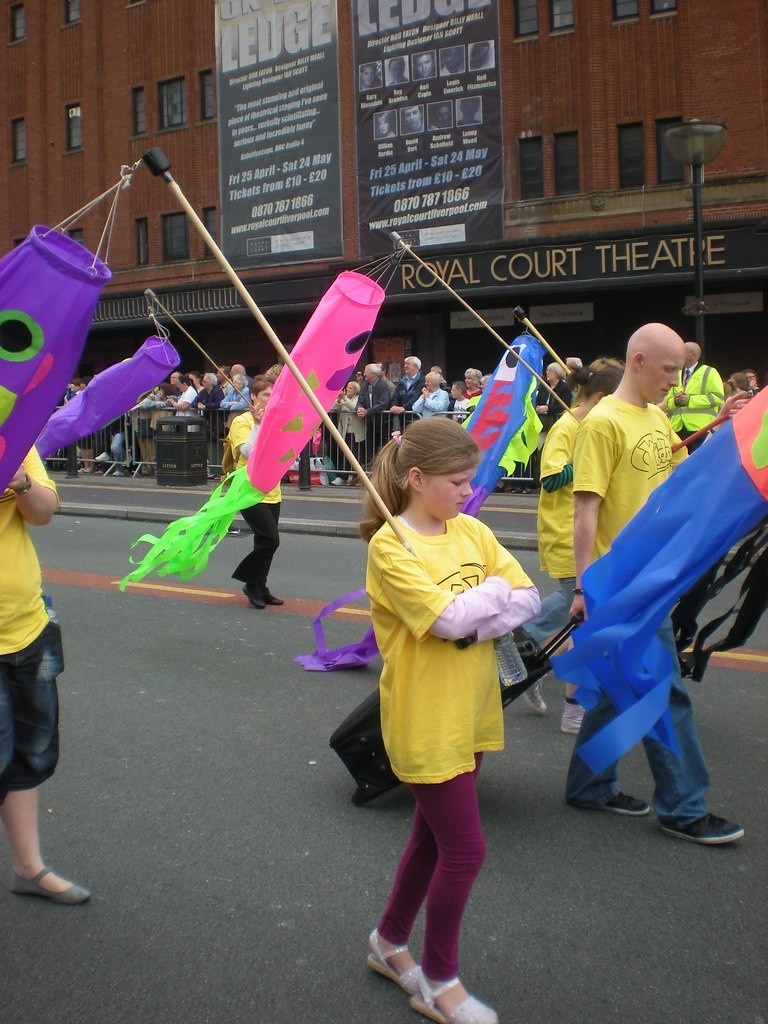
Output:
[329,611,583,806]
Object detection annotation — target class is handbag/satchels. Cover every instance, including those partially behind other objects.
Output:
[288,457,337,486]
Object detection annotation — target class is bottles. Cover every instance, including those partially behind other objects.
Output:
[489,630,529,688]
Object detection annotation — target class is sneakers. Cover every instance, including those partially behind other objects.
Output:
[656,813,745,844]
[346,475,357,486]
[599,792,650,815]
[523,680,547,713]
[561,700,585,734]
[95,452,110,461]
[331,477,347,486]
[112,471,125,475]
[77,468,94,473]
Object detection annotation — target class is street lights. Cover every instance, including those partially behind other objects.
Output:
[662,116,730,352]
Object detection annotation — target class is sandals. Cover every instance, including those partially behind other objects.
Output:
[366,929,422,995]
[410,972,499,1024]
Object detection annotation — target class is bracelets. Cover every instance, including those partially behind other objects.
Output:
[15,474,32,495]
[710,428,716,434]
[572,588,584,594]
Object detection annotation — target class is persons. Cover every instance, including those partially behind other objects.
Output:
[442,47,464,74]
[457,98,482,125]
[363,418,542,1024]
[565,322,744,841]
[469,42,492,69]
[227,364,289,609]
[375,111,396,137]
[51,363,253,481]
[414,52,436,79]
[652,342,760,456]
[388,57,409,84]
[317,356,583,494]
[0,443,92,906]
[360,62,382,89]
[403,107,424,133]
[520,358,625,733]
[429,102,452,129]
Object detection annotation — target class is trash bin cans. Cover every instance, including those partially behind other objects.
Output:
[153,415,209,487]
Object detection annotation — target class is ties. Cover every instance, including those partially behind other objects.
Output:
[685,370,690,386]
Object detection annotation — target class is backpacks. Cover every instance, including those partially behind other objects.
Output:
[218,415,251,485]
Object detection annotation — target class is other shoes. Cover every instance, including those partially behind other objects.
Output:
[10,868,90,904]
[243,584,284,609]
[522,487,532,493]
[511,487,524,493]
